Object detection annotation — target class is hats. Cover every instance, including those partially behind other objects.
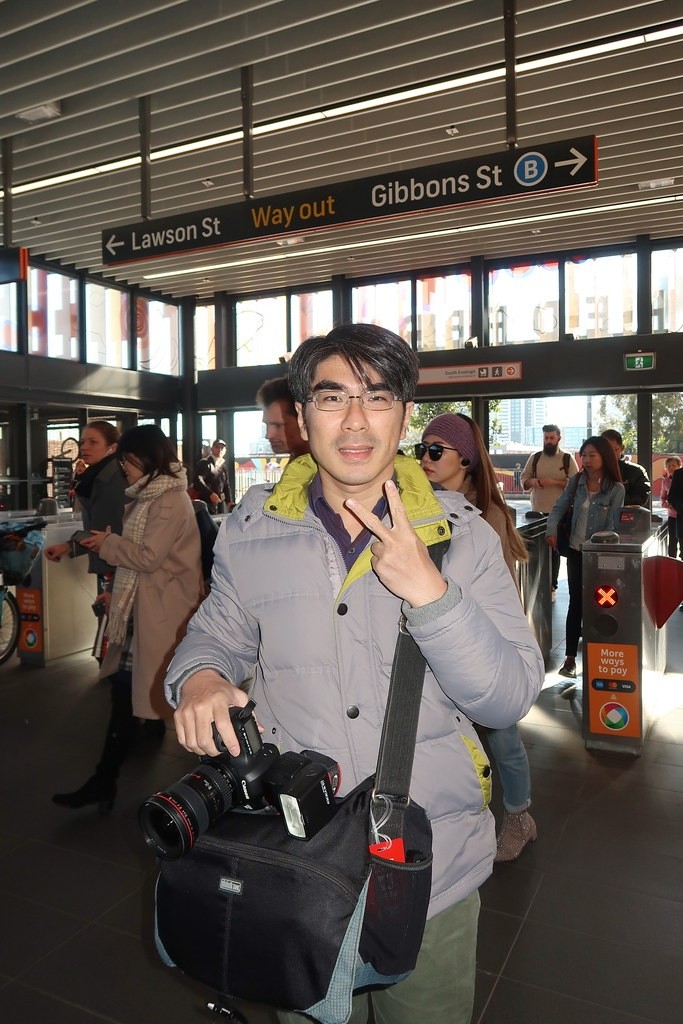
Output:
[213,439,226,446]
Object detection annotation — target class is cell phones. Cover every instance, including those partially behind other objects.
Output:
[71,530,96,542]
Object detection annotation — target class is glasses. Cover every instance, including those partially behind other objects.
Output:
[413,442,457,460]
[304,388,404,412]
[119,456,137,469]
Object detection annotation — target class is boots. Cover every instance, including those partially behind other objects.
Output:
[52,686,132,815]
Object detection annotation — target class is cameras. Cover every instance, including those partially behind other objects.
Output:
[137,705,341,864]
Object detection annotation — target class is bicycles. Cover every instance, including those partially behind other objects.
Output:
[0,521,49,665]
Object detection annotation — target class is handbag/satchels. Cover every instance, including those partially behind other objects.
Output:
[151,773,431,1009]
[190,500,220,580]
[556,470,583,556]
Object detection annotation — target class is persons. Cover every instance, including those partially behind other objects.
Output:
[194,439,234,515]
[415,413,683,603]
[45,420,135,668]
[546,436,626,678]
[164,324,545,1024]
[51,425,205,813]
[255,377,312,463]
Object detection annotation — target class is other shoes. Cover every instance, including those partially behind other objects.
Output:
[559,658,577,678]
[496,811,539,861]
